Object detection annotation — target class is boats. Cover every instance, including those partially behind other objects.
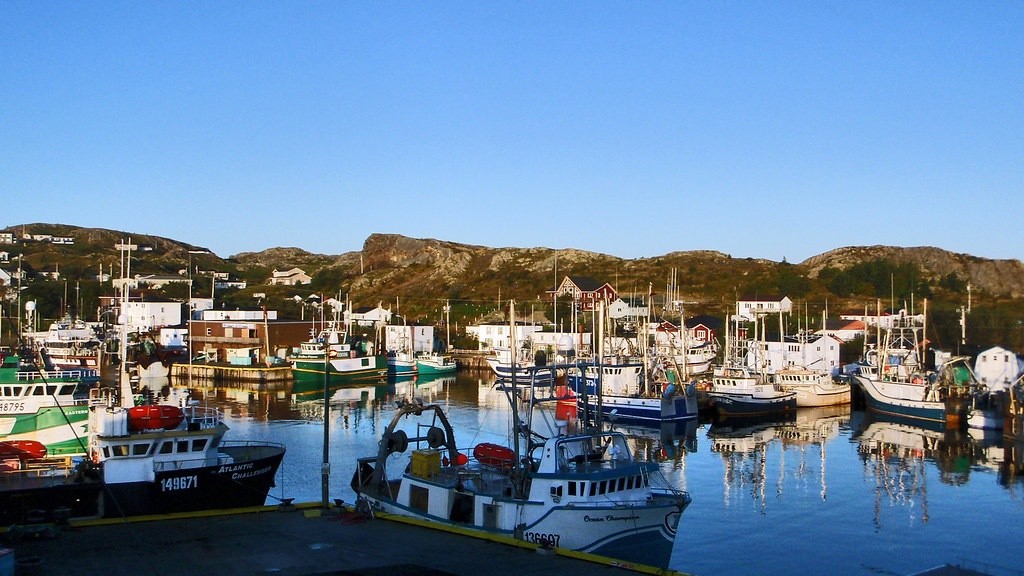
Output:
[358,351,691,575]
[3,308,285,518]
[280,290,1022,444]
